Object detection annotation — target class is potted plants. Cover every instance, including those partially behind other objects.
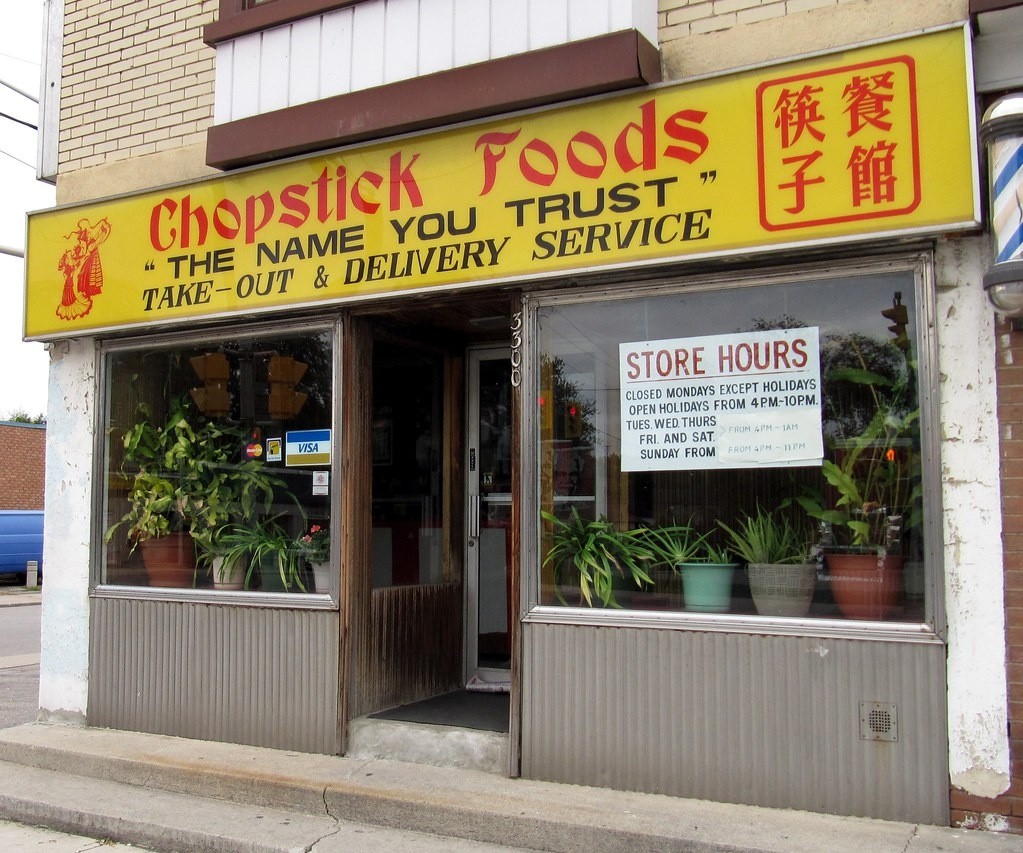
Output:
[107,411,288,590]
[573,534,659,611]
[221,522,313,595]
[199,528,251,590]
[643,516,735,613]
[721,500,827,615]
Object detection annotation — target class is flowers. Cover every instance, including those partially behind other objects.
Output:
[301,525,332,565]
[763,322,923,557]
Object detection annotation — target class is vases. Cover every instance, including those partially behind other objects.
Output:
[825,550,904,620]
[313,562,332,592]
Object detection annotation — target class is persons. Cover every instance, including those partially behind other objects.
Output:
[569,439,596,529]
